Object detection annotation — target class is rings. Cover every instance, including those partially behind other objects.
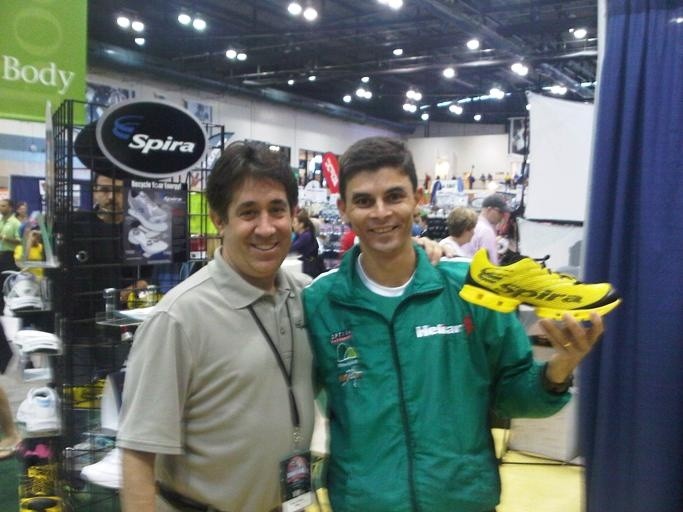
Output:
[561,340,573,348]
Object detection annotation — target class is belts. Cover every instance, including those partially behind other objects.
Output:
[154,482,217,512]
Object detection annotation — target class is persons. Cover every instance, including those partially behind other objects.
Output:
[13,222,45,284]
[119,263,152,309]
[0,321,23,461]
[90,173,122,233]
[298,136,604,511]
[0,199,24,315]
[111,141,455,512]
[14,202,30,230]
[289,194,513,277]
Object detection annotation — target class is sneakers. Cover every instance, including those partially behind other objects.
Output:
[17,388,63,436]
[120,283,164,341]
[3,316,62,355]
[62,379,122,490]
[458,246,623,324]
[13,438,63,512]
[0,269,44,311]
[125,189,169,255]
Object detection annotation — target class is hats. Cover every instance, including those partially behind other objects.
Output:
[20,222,38,237]
[482,195,515,212]
